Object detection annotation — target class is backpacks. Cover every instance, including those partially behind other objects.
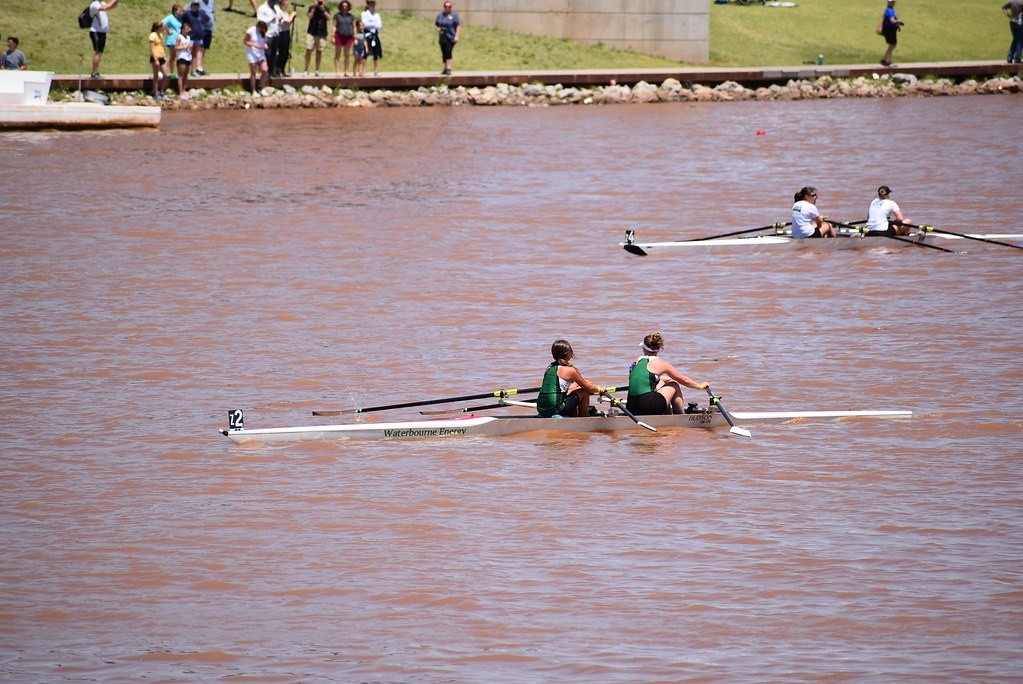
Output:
[78,2,99,29]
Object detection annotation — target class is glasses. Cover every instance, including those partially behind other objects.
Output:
[810,194,817,197]
[444,5,451,8]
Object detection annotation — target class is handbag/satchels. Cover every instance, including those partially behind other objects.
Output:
[877,20,884,37]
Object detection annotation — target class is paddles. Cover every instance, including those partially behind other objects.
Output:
[826,218,959,253]
[705,384,754,439]
[311,386,542,417]
[604,390,657,433]
[842,216,890,226]
[419,384,629,416]
[672,215,827,242]
[901,222,1023,250]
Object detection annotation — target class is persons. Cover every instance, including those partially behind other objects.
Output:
[161,0,215,101]
[625,332,710,415]
[303,0,332,77]
[537,340,607,416]
[353,21,368,77]
[331,0,359,78]
[880,0,904,67]
[244,21,268,97]
[222,0,259,18]
[257,0,297,76]
[434,0,460,74]
[89,0,118,78]
[865,186,912,235]
[0,33,27,71]
[359,0,382,76]
[1001,0,1023,63]
[792,187,835,238]
[149,22,169,100]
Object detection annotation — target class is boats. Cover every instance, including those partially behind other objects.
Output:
[215,409,915,445]
[619,229,1023,246]
[0,98,161,130]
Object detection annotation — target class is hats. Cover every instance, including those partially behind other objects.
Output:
[191,0,201,5]
[888,0,894,3]
[878,186,893,195]
[367,0,375,4]
[638,340,660,352]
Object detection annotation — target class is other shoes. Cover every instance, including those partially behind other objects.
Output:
[169,72,177,79]
[315,71,323,77]
[252,93,260,98]
[179,94,188,100]
[880,60,889,66]
[185,92,192,99]
[303,71,308,76]
[442,67,451,75]
[344,73,351,78]
[336,73,341,77]
[153,92,163,100]
[1008,58,1013,63]
[91,72,102,79]
[191,72,200,77]
[1016,60,1022,62]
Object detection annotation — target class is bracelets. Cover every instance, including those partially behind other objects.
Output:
[323,9,326,12]
[594,387,600,394]
[897,21,898,23]
